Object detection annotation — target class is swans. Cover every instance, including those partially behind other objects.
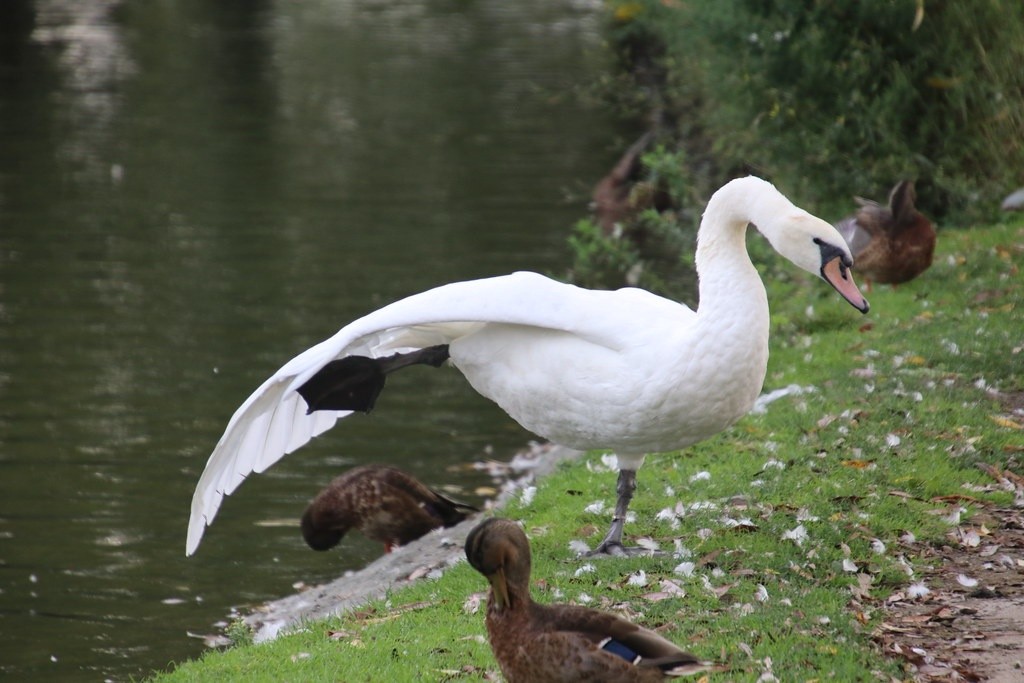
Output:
[185,174,871,562]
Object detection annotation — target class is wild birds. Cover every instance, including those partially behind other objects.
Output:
[584,124,936,294]
[301,465,730,683]
